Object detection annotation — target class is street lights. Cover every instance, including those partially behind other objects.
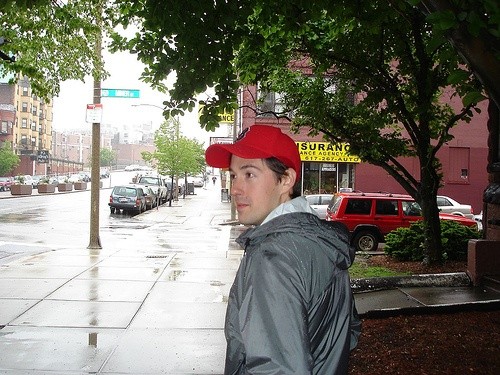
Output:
[132,102,178,207]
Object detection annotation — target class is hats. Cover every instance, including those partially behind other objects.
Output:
[206,125,301,181]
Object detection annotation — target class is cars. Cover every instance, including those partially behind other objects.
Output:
[109,184,146,215]
[29,175,49,188]
[174,176,187,196]
[0,176,15,191]
[191,173,209,187]
[47,175,67,186]
[14,174,34,189]
[127,183,158,209]
[68,169,110,183]
[125,164,155,171]
[162,177,179,198]
[416,194,475,222]
[305,193,333,220]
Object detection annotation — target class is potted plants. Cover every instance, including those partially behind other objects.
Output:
[58,176,73,191]
[10,172,32,196]
[74,179,88,190]
[37,176,55,193]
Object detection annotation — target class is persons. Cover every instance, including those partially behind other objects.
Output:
[206,126,362,375]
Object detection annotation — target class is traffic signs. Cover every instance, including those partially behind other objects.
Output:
[100,87,141,98]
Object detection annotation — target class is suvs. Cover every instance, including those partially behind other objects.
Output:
[325,190,480,252]
[135,176,168,204]
